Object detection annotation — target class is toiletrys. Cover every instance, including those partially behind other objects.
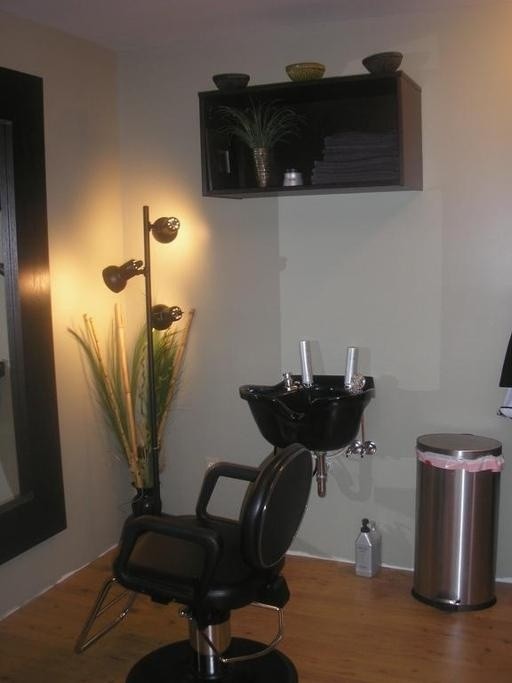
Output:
[353,516,382,580]
[367,520,382,569]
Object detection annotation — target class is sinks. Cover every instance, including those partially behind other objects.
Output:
[237,374,376,451]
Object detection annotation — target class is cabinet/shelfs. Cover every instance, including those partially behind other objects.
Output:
[197,69,424,198]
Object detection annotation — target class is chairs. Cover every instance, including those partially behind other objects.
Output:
[76,441,314,683]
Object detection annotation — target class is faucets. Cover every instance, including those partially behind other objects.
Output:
[281,368,298,392]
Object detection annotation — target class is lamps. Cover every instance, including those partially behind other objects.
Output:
[102,203,182,515]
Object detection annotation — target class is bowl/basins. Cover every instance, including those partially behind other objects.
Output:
[286,62,326,81]
[212,73,251,90]
[361,51,403,73]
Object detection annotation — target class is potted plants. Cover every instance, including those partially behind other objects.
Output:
[215,97,299,188]
[69,300,195,514]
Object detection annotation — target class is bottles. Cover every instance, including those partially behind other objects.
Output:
[282,167,303,187]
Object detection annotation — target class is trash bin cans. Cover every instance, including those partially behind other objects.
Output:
[412,433,503,612]
